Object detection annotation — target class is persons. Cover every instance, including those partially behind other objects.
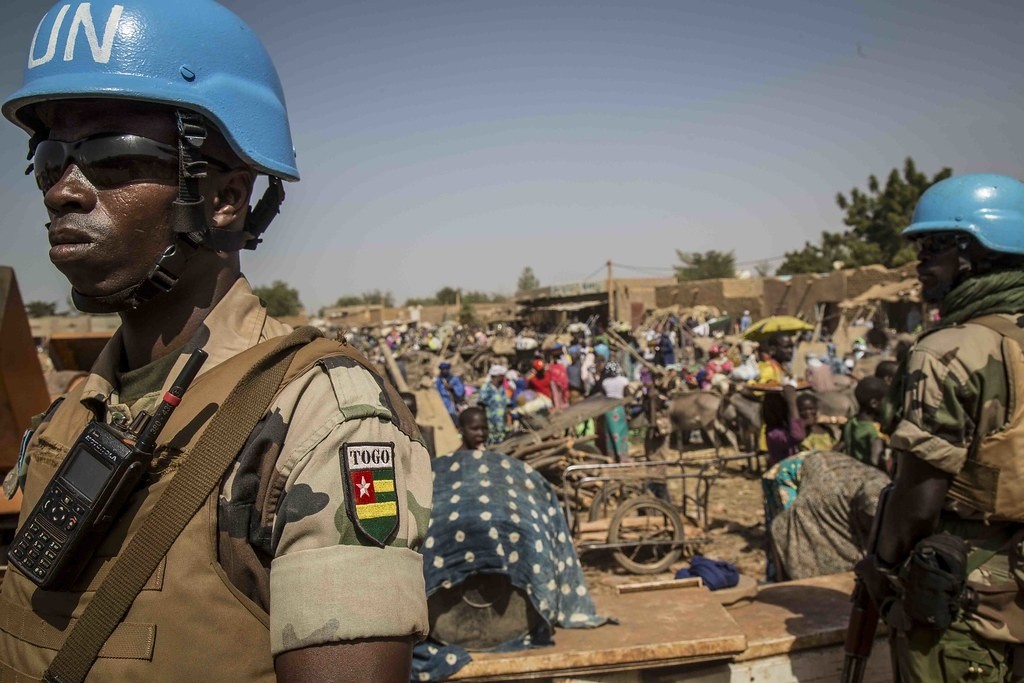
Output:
[855,173,1024,683]
[344,302,922,579]
[0,0,433,683]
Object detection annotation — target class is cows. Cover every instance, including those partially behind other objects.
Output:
[658,390,856,461]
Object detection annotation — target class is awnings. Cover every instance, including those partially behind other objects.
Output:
[531,300,605,311]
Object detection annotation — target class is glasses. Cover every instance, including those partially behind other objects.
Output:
[917,232,974,254]
[35,131,234,191]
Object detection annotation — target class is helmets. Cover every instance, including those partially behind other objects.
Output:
[901,172,1024,255]
[0,0,300,182]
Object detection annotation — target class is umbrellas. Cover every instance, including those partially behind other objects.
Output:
[743,314,814,343]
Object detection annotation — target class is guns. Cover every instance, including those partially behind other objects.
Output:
[841,449,922,683]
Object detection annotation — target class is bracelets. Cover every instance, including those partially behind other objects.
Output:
[876,551,896,569]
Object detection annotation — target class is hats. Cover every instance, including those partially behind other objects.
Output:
[490,365,507,376]
[439,362,451,369]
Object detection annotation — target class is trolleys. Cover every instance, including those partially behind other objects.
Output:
[561,452,763,574]
